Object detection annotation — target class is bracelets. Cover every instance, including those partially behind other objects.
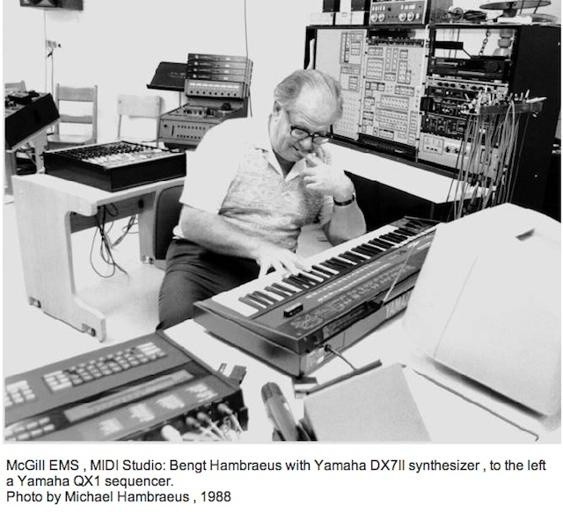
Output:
[333,190,357,208]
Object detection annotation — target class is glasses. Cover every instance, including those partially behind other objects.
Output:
[287,112,333,145]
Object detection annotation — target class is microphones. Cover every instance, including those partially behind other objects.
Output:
[261,382,300,441]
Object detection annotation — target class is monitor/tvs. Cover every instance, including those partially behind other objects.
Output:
[396,201,561,438]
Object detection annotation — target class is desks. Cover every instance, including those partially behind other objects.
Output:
[9,142,187,342]
[161,217,561,443]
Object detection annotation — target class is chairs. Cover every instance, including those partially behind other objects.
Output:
[46,83,162,150]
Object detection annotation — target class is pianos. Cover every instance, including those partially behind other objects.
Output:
[193,216,442,376]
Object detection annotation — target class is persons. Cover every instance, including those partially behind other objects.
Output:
[157,70,367,331]
